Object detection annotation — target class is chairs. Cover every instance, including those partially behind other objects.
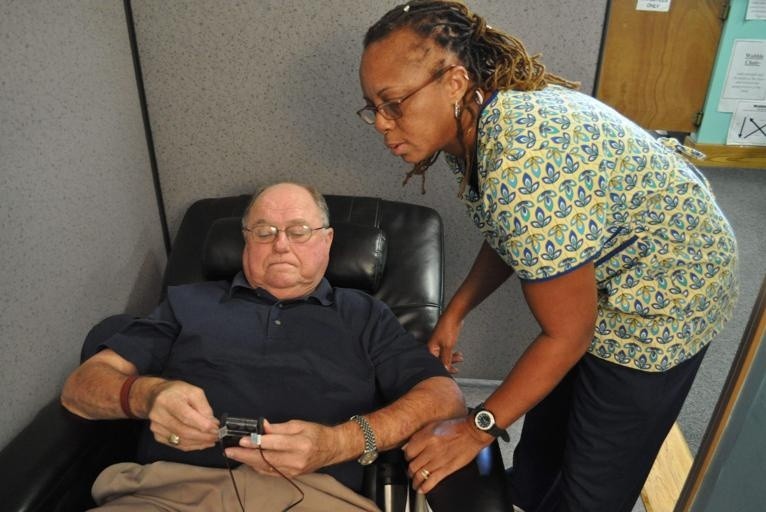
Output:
[0,193,443,511]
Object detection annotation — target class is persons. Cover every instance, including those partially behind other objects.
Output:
[58,181,468,512]
[357,1,739,512]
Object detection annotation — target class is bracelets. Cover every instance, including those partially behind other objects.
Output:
[120,375,139,422]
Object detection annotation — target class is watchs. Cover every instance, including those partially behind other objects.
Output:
[349,413,378,467]
[474,402,510,443]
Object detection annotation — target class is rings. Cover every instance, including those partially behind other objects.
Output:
[266,466,274,474]
[419,467,430,479]
[168,432,180,446]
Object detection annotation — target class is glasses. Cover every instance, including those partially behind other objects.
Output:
[354,62,458,125]
[239,223,328,245]
